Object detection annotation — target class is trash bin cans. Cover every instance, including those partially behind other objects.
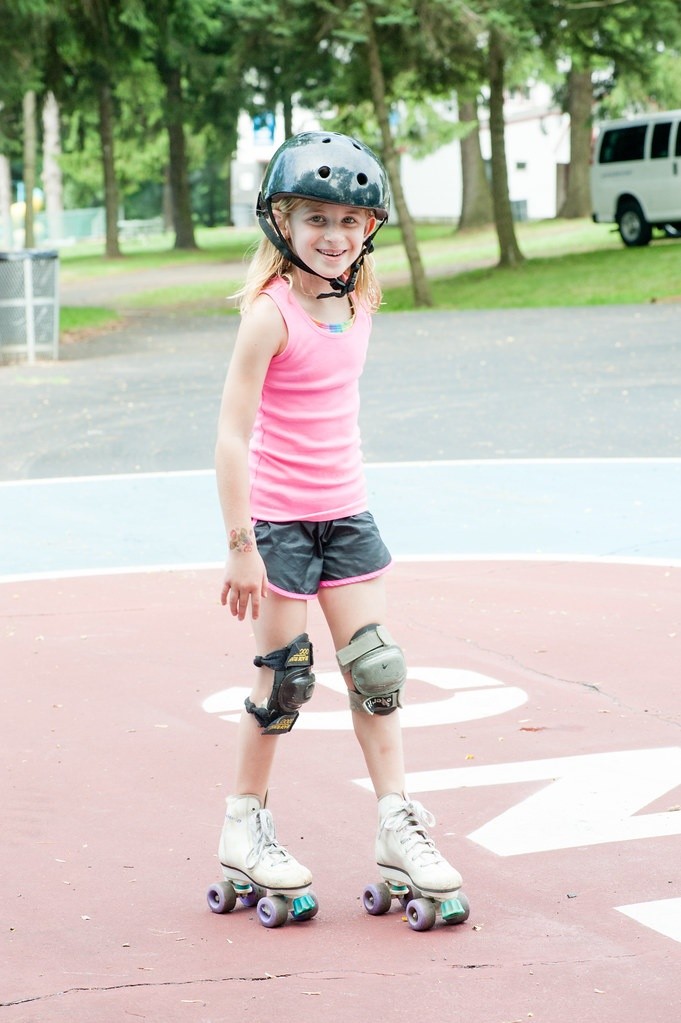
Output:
[0,251,61,364]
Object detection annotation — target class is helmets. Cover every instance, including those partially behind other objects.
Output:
[259,131,390,220]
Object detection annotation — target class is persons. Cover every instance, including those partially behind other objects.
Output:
[210,130,471,932]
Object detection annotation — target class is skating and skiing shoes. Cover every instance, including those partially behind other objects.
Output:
[206,794,319,928]
[361,790,469,929]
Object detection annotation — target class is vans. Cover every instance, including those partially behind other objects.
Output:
[588,110,681,249]
